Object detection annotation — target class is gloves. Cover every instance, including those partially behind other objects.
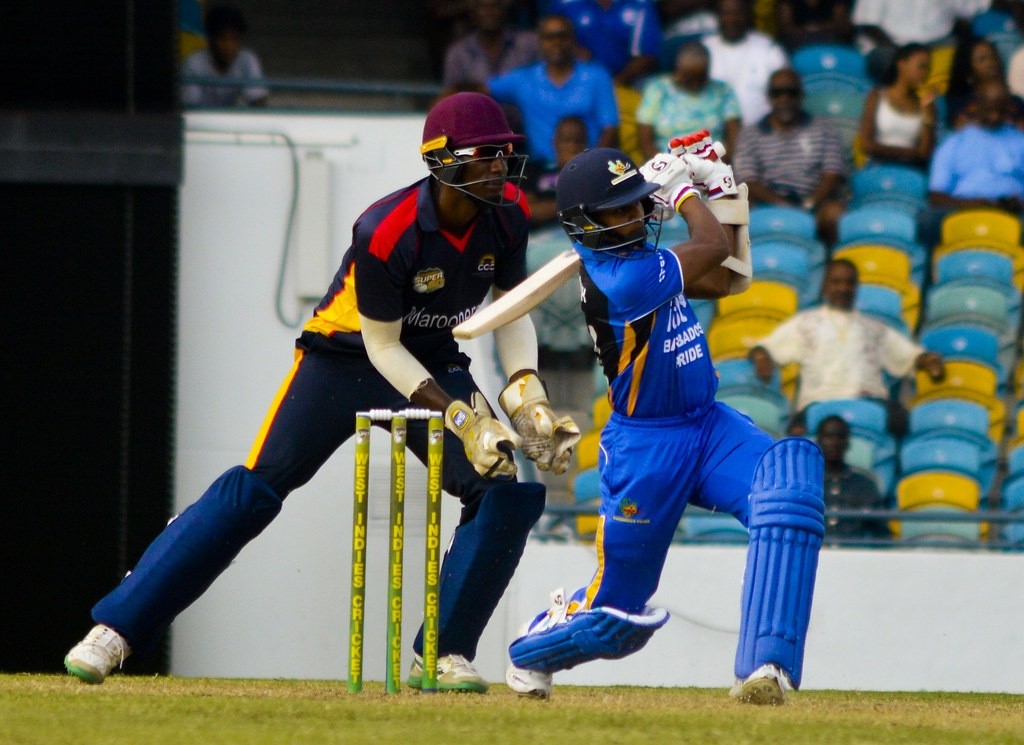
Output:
[638,154,700,214]
[445,392,522,484]
[668,129,738,200]
[498,375,580,478]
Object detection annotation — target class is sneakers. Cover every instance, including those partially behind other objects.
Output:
[729,660,784,706]
[407,651,486,695]
[506,607,551,702]
[64,620,131,686]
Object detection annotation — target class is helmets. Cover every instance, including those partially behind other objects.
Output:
[554,147,660,252]
[422,92,527,187]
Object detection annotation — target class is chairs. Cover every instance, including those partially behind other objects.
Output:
[524,11,1024,547]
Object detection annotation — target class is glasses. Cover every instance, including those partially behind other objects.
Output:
[452,143,513,162]
[541,30,565,42]
[768,86,797,99]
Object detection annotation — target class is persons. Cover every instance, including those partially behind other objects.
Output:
[178,17,268,110]
[811,415,892,543]
[480,0,657,229]
[446,0,543,93]
[504,129,824,707]
[637,0,843,246]
[749,259,946,480]
[62,92,581,692]
[852,0,1023,257]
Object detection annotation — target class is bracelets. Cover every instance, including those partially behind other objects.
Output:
[757,356,767,360]
